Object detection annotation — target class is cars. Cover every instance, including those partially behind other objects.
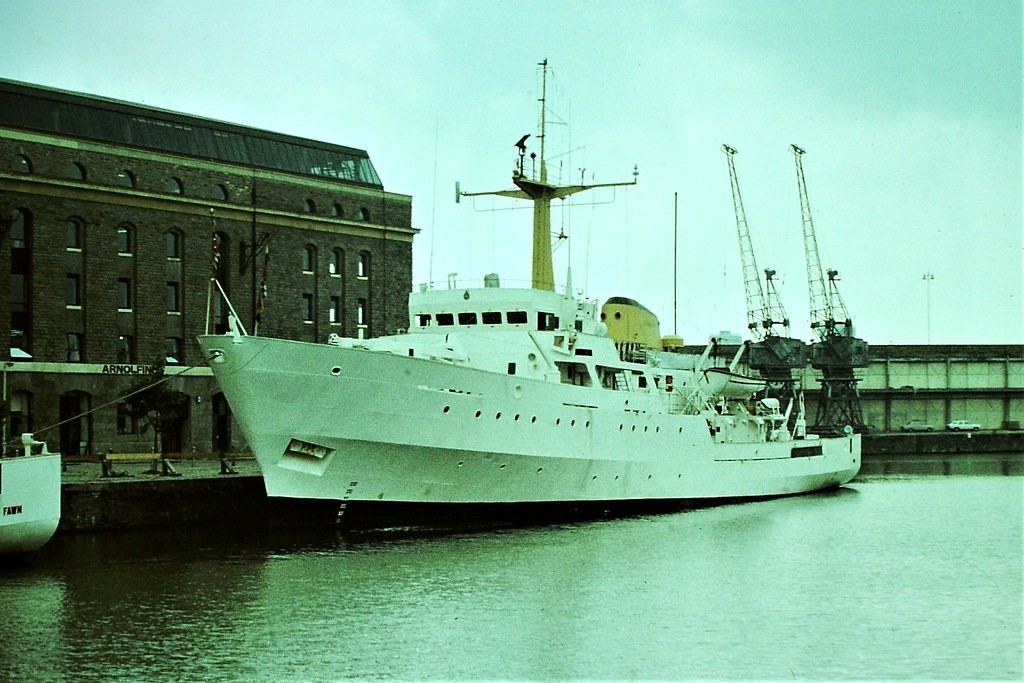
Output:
[946,421,982,433]
[901,419,936,433]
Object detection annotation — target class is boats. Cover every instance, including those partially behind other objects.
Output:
[0,433,61,559]
[196,262,862,529]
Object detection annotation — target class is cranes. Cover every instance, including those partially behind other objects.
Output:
[717,144,812,428]
[788,143,874,432]
